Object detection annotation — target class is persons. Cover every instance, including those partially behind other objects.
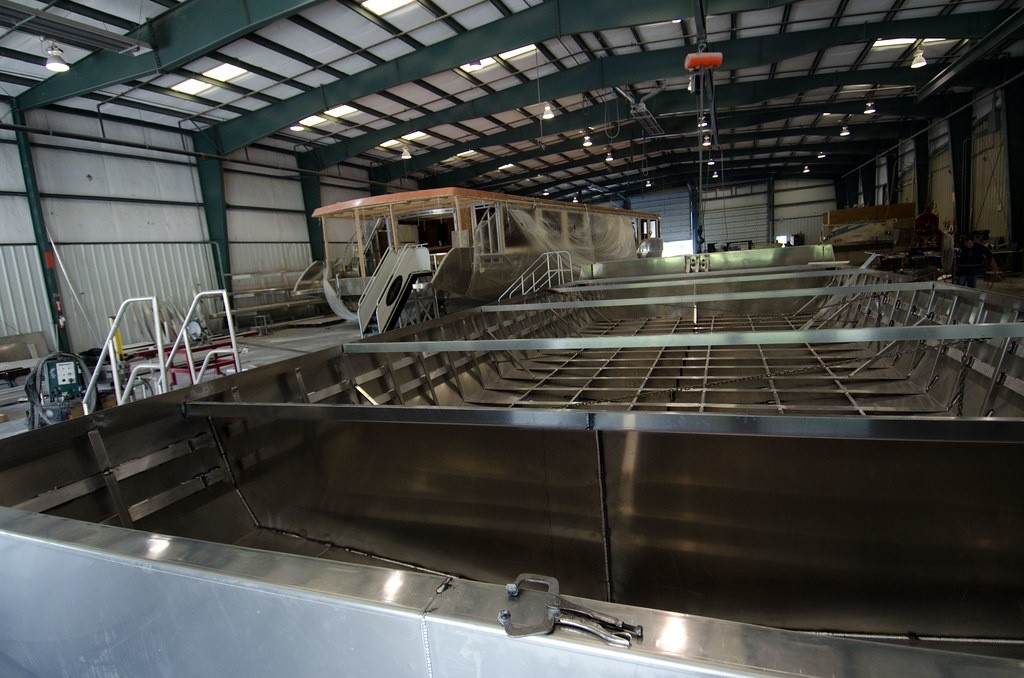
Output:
[956,236,996,288]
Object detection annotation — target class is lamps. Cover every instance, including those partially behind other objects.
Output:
[708,158,715,166]
[911,45,927,68]
[702,134,712,147]
[698,114,708,128]
[818,150,827,159]
[583,134,593,148]
[606,152,613,163]
[543,106,555,120]
[401,145,413,160]
[840,123,850,137]
[863,100,876,115]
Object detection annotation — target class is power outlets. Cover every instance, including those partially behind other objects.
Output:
[56,361,77,386]
